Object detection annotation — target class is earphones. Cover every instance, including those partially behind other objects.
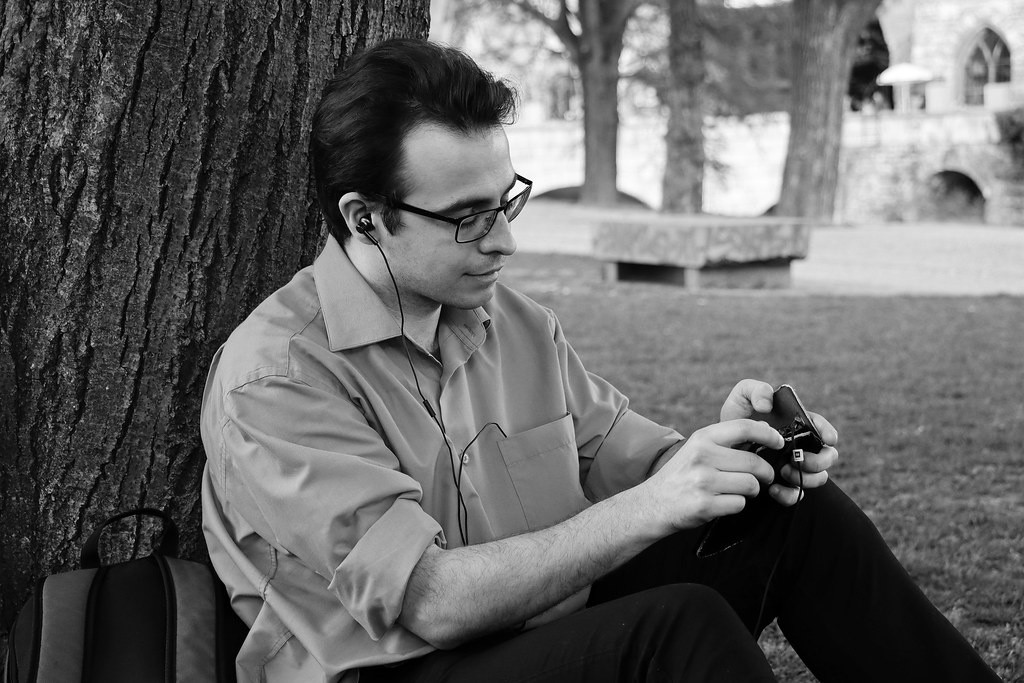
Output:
[355,212,379,244]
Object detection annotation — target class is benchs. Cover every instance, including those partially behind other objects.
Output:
[506,201,814,292]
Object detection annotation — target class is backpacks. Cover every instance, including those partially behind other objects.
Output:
[2,507,249,683]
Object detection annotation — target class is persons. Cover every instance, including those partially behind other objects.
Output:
[198,38,1003,683]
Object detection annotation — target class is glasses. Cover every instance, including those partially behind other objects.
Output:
[367,172,533,243]
